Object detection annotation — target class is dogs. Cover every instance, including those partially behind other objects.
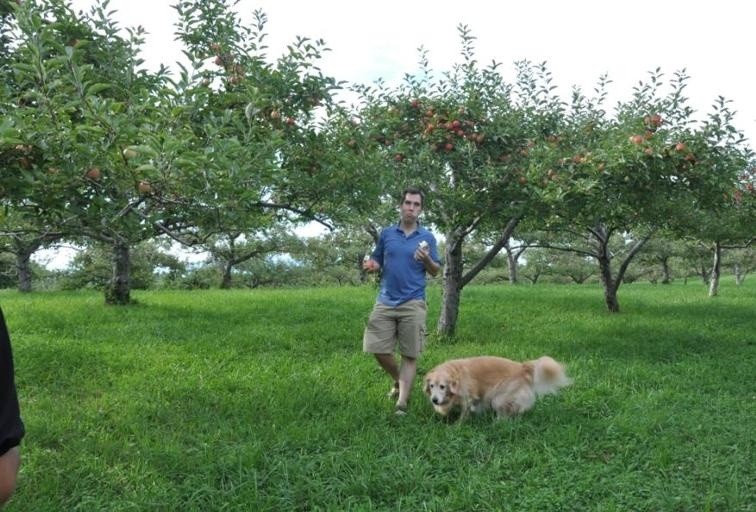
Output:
[422,355,570,427]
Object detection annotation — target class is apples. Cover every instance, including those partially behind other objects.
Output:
[0,1,752,201]
[366,260,374,270]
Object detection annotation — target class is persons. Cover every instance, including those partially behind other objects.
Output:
[1,308,25,504]
[363,188,441,414]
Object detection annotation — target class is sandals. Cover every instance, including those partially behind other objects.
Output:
[388,382,408,416]
